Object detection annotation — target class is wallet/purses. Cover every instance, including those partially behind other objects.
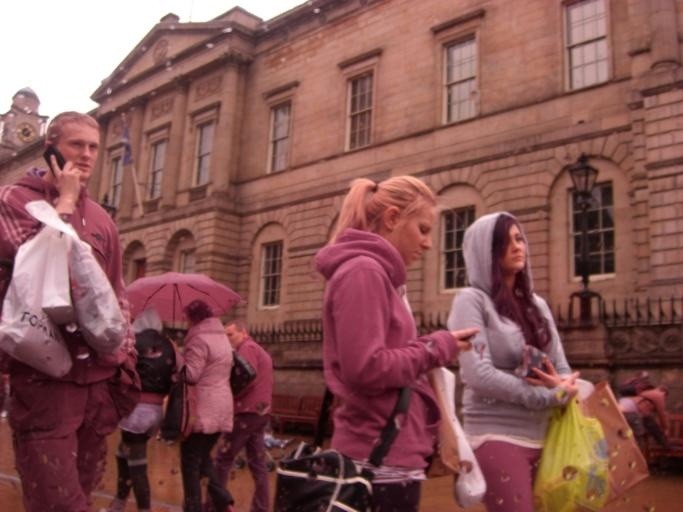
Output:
[515,345,552,378]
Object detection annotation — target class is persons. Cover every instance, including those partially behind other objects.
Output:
[315,173,481,512]
[618,371,673,464]
[449,210,580,512]
[0,111,141,512]
[101,300,274,512]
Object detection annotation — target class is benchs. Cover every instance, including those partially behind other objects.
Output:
[268,394,335,441]
[647,413,683,470]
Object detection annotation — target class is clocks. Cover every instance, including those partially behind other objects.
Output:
[16,122,37,145]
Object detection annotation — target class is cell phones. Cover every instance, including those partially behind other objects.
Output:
[43,144,66,176]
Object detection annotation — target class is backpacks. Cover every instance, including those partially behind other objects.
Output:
[133,329,178,393]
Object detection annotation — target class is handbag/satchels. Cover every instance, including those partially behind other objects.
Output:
[272,441,373,511]
[575,380,654,507]
[160,362,190,443]
[228,351,256,396]
[0,199,129,381]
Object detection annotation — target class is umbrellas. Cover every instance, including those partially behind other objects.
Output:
[124,271,242,340]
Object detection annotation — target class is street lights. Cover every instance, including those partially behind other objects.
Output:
[560,149,611,321]
